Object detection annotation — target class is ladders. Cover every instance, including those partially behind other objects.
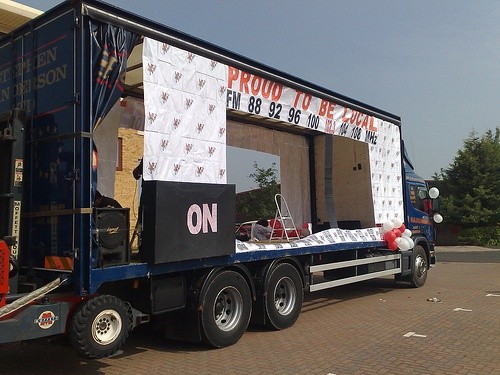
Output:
[268,193,300,243]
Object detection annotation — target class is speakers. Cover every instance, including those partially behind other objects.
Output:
[94,207,131,267]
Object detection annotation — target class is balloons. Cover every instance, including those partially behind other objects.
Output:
[395,237,410,251]
[388,241,398,250]
[391,218,402,228]
[401,235,414,249]
[433,213,443,223]
[393,228,401,237]
[383,220,394,231]
[398,224,405,233]
[429,187,439,198]
[401,229,412,237]
[383,231,396,242]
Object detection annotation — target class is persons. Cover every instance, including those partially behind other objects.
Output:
[253,218,275,241]
[95,190,123,208]
[131,155,143,262]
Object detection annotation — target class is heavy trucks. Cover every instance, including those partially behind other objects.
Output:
[0,2,443,348]
